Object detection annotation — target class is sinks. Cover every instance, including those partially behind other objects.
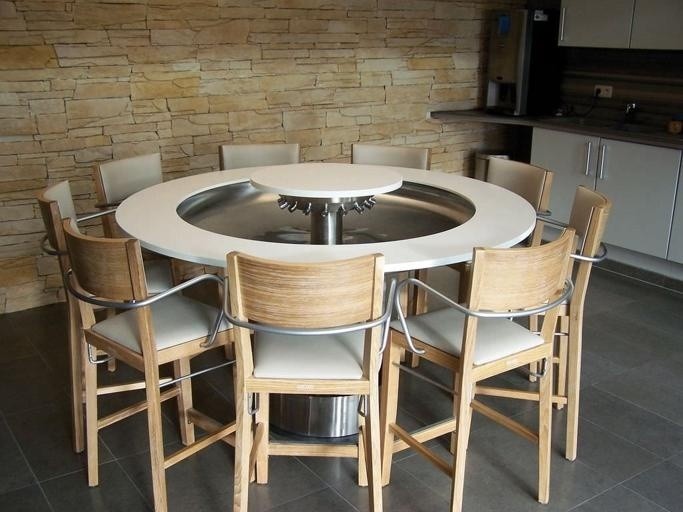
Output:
[536,112,665,139]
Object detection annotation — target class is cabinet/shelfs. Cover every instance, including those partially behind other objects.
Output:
[530,121,683,280]
[558,0,683,50]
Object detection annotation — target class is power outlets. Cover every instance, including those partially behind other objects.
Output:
[593,84,613,98]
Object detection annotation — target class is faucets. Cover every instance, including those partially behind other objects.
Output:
[624,102,636,116]
